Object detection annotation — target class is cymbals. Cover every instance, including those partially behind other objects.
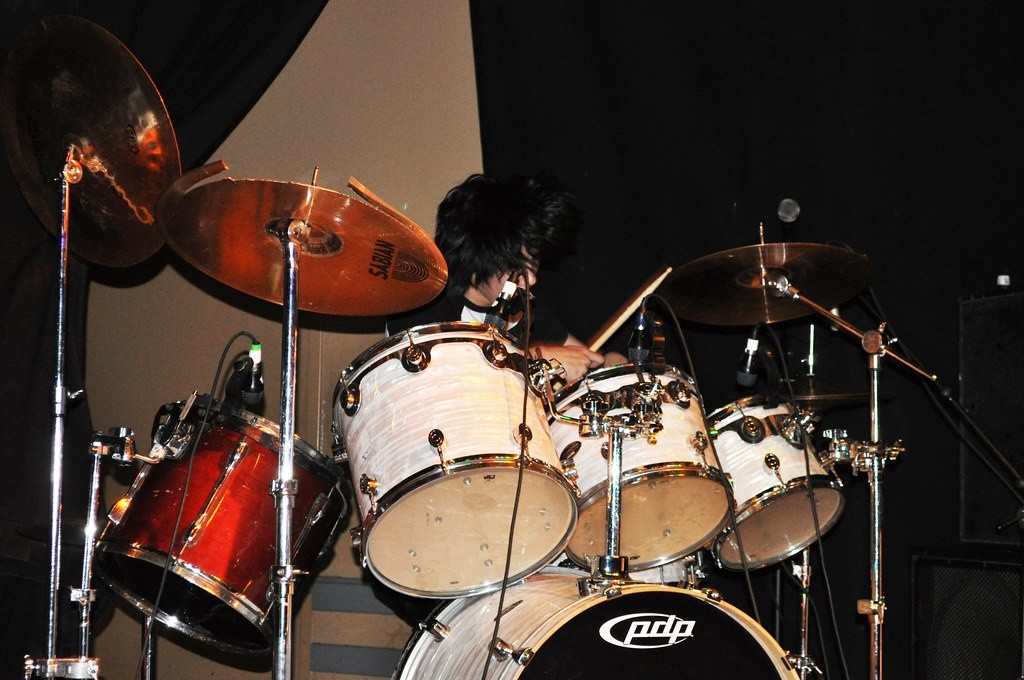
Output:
[650,244,875,326]
[778,385,872,407]
[2,14,179,270]
[14,520,107,547]
[155,159,449,318]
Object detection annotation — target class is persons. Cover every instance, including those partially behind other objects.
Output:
[433,172,628,384]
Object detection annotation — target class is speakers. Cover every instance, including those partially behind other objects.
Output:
[908,547,1024,680]
[958,290,1024,546]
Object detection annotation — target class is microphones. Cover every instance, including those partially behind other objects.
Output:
[736,324,761,386]
[484,270,520,330]
[242,344,263,406]
[627,304,650,364]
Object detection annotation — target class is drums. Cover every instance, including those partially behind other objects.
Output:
[707,391,845,571]
[545,361,736,574]
[389,568,802,680]
[332,321,580,600]
[92,393,352,658]
